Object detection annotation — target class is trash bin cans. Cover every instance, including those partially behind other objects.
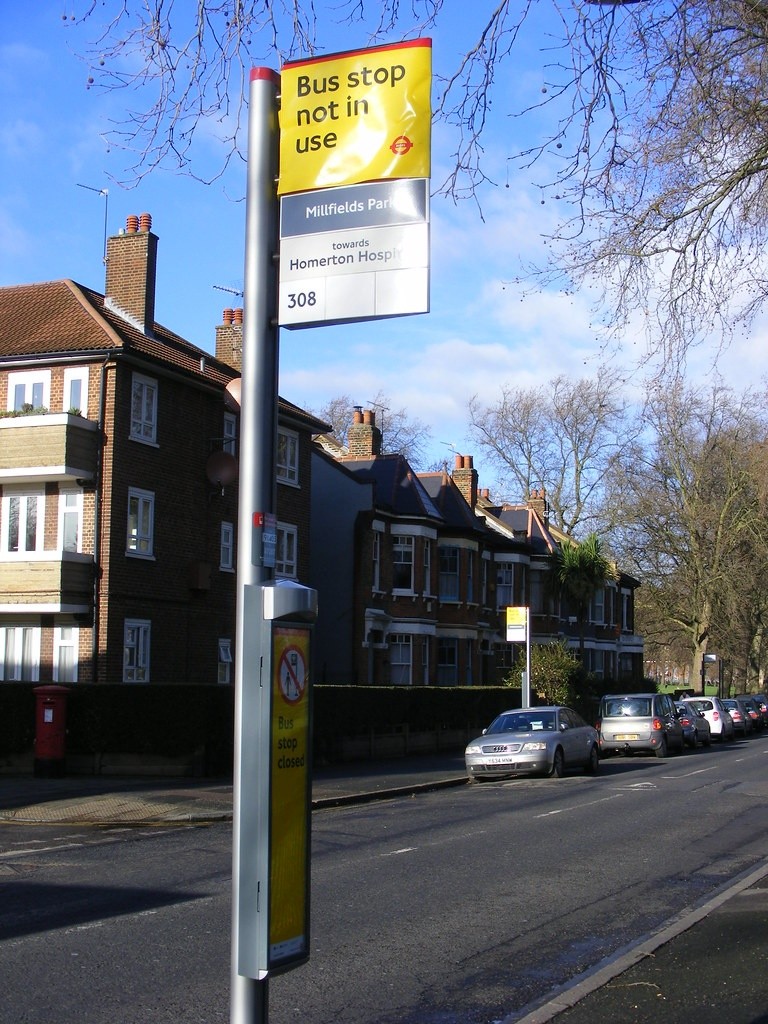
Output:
[33,685,71,762]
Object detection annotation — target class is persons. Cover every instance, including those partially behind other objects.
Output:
[680,692,690,702]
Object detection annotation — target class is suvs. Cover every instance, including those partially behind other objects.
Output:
[594,692,685,758]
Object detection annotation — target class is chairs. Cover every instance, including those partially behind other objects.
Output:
[514,719,530,731]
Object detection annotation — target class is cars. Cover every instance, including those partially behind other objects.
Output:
[736,693,768,734]
[721,699,754,737]
[682,697,735,744]
[733,697,762,734]
[672,700,712,750]
[465,707,601,784]
